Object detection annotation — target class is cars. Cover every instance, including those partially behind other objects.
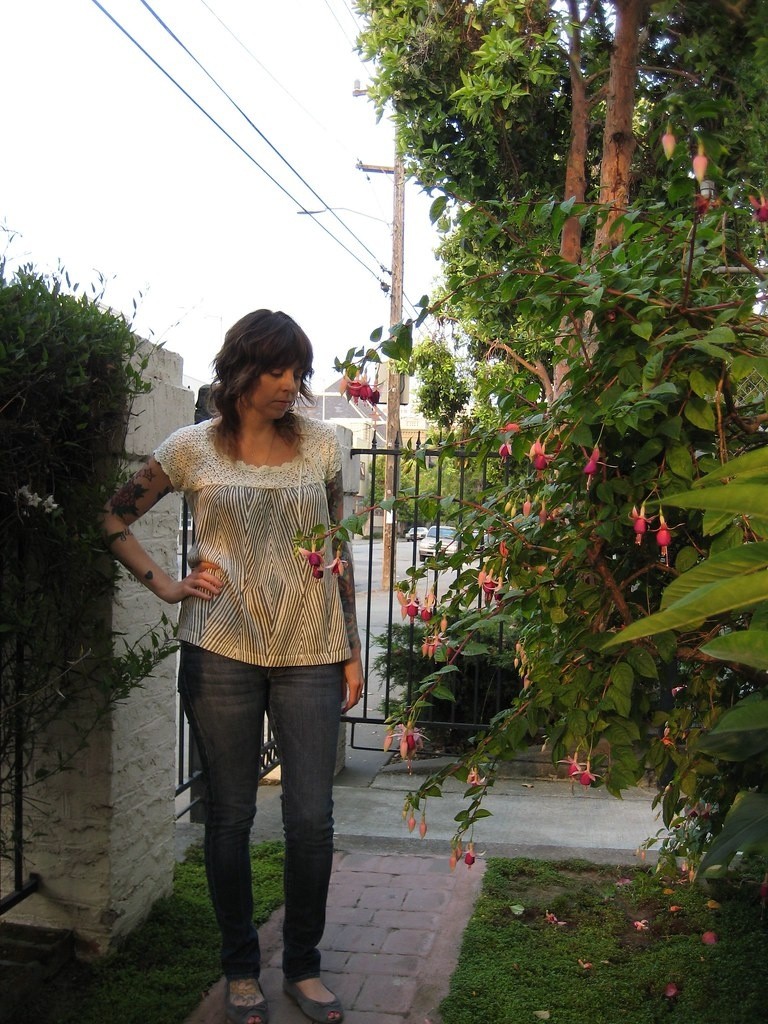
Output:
[418,526,459,561]
[470,529,496,552]
[406,527,429,542]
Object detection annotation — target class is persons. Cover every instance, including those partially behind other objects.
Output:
[97,310,364,1024]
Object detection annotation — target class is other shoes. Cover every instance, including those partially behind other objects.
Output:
[224,979,269,1024]
[283,977,344,1024]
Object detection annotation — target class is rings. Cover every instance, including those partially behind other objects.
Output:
[360,695,363,698]
